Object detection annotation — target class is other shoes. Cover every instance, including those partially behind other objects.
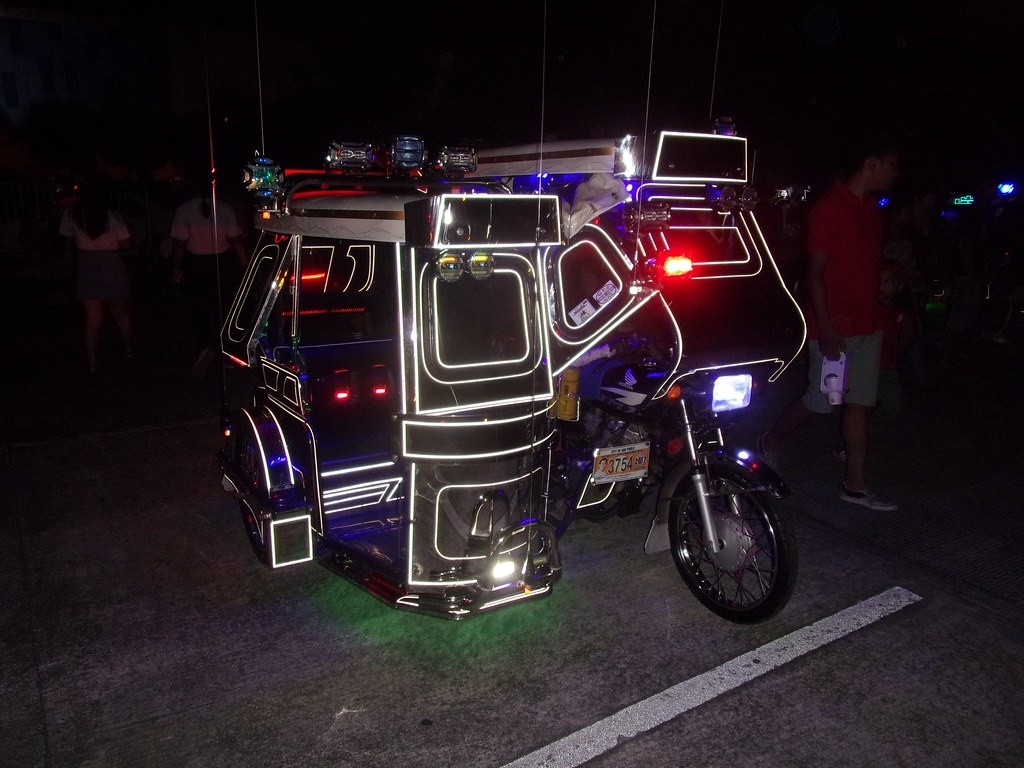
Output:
[831,446,846,459]
[840,482,900,511]
[192,347,216,378]
[756,431,784,470]
[87,363,97,374]
[126,353,135,360]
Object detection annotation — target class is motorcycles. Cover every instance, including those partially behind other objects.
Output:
[218,116,808,625]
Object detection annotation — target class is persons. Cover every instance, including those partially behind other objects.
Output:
[59,173,141,372]
[756,138,902,512]
[169,171,249,382]
